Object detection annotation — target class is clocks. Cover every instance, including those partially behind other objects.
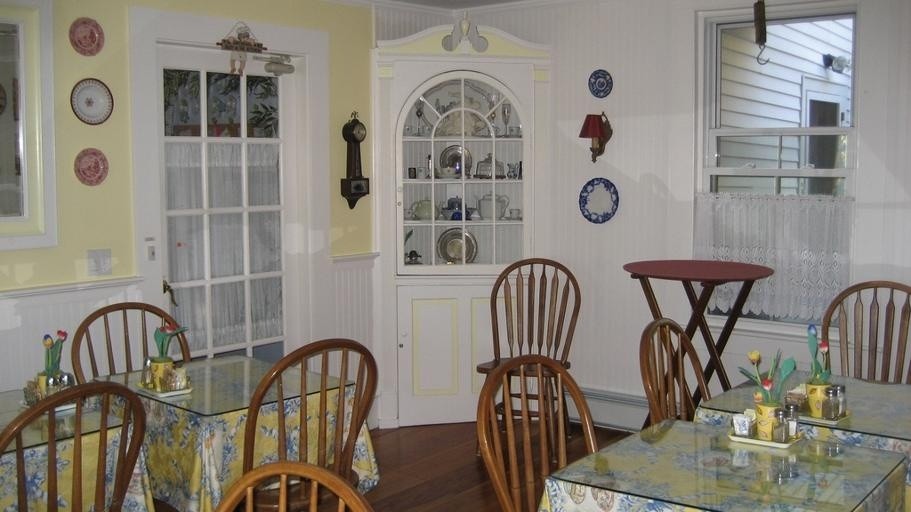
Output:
[340,111,370,209]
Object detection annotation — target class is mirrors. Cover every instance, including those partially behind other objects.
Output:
[1,22,29,223]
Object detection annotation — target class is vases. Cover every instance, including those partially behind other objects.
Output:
[757,403,783,440]
[37,372,56,396]
[805,382,832,419]
[151,362,173,391]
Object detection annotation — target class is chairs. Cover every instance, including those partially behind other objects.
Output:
[477,258,580,458]
[640,319,712,424]
[476,354,598,512]
[821,281,911,384]
[72,303,190,388]
[215,461,375,512]
[241,338,378,512]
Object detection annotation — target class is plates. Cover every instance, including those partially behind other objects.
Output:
[71,79,116,125]
[588,70,612,98]
[74,148,110,186]
[440,145,472,177]
[68,18,105,57]
[579,177,619,224]
[504,217,522,220]
[436,227,477,264]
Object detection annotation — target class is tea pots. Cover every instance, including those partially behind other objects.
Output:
[507,162,520,179]
[410,197,440,219]
[473,191,509,220]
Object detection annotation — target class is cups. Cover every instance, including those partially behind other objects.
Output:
[409,168,416,178]
[404,125,412,136]
[489,127,501,137]
[510,127,520,136]
[404,209,412,219]
[510,209,519,218]
[417,167,429,179]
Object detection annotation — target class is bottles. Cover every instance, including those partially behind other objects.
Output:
[822,388,840,420]
[785,404,798,440]
[832,384,847,418]
[772,408,789,443]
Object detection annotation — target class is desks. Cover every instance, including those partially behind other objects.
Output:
[623,259,774,431]
[538,420,909,512]
[694,366,910,511]
[87,356,381,512]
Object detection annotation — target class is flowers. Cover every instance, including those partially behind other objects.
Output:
[738,350,795,405]
[154,322,189,358]
[807,323,831,384]
[45,331,67,374]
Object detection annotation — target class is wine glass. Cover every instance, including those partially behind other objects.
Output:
[501,104,511,137]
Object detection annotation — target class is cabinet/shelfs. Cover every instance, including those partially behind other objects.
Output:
[365,15,554,430]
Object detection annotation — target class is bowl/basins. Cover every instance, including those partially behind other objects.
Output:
[438,207,478,220]
